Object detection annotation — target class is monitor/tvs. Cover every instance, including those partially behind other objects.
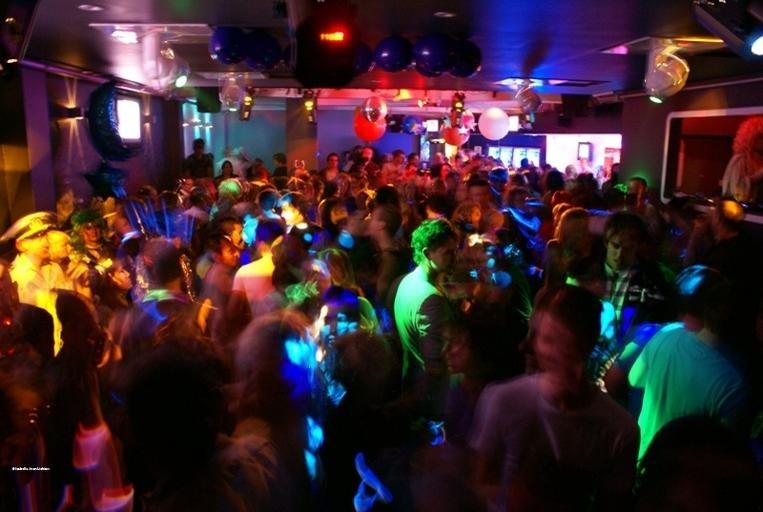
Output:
[111,94,145,149]
[577,142,592,161]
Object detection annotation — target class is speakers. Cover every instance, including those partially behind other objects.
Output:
[194,87,220,113]
[560,94,590,118]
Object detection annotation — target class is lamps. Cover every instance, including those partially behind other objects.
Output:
[745,28,763,56]
[238,94,254,120]
[451,90,466,117]
[641,42,691,104]
[303,91,318,125]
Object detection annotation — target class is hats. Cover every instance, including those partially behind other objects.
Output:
[0,210,57,243]
